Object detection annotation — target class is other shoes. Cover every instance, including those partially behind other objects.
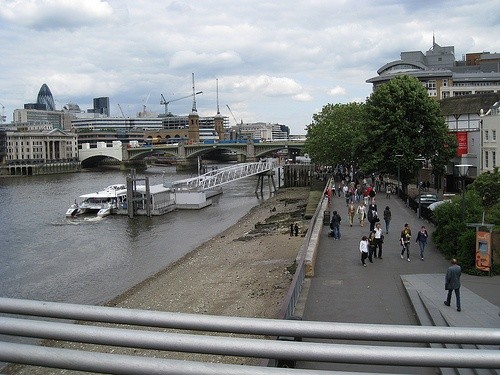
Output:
[370,260,373,263]
[374,256,377,259]
[444,301,449,306]
[400,254,404,258]
[457,309,461,312]
[363,264,367,266]
[334,238,338,240]
[407,258,410,262]
[422,258,424,260]
[378,257,383,259]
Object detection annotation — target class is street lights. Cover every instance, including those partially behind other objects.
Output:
[454,164,475,222]
[414,158,425,217]
[395,154,404,198]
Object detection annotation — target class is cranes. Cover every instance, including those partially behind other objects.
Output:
[159,91,204,114]
[225,103,243,132]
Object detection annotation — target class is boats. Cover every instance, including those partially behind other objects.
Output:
[66,184,131,218]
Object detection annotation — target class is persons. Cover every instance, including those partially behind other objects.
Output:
[332,181,396,231]
[416,226,428,261]
[373,223,384,259]
[384,206,391,234]
[368,232,376,263]
[419,180,430,192]
[360,235,369,267]
[444,259,461,312]
[400,223,412,262]
[331,211,341,240]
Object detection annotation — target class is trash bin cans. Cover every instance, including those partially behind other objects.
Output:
[324,210,330,224]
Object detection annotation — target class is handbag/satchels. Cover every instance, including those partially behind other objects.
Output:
[376,217,380,222]
[364,213,366,218]
[445,282,451,290]
